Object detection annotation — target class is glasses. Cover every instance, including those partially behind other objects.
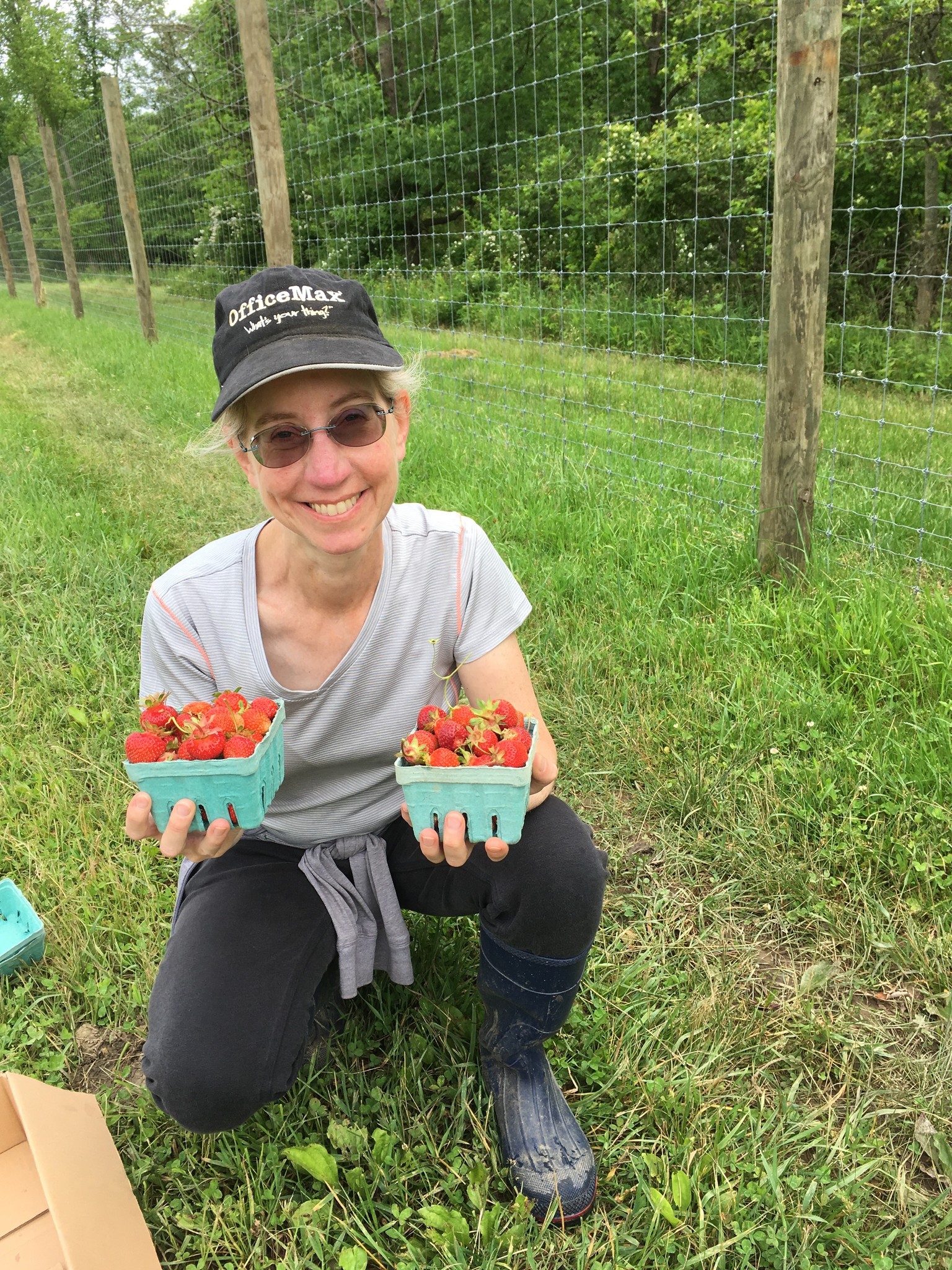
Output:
[234,398,395,469]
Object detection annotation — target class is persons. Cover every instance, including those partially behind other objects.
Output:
[126,265,608,1224]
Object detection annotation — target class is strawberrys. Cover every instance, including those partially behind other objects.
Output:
[125,686,279,824]
[399,698,533,768]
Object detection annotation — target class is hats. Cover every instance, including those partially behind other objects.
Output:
[209,265,405,425]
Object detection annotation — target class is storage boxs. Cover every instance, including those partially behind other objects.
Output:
[0,878,47,981]
[395,716,536,844]
[119,697,286,835]
[0,1070,165,1270]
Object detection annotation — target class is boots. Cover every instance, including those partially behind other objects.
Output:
[303,957,349,1072]
[471,918,598,1224]
